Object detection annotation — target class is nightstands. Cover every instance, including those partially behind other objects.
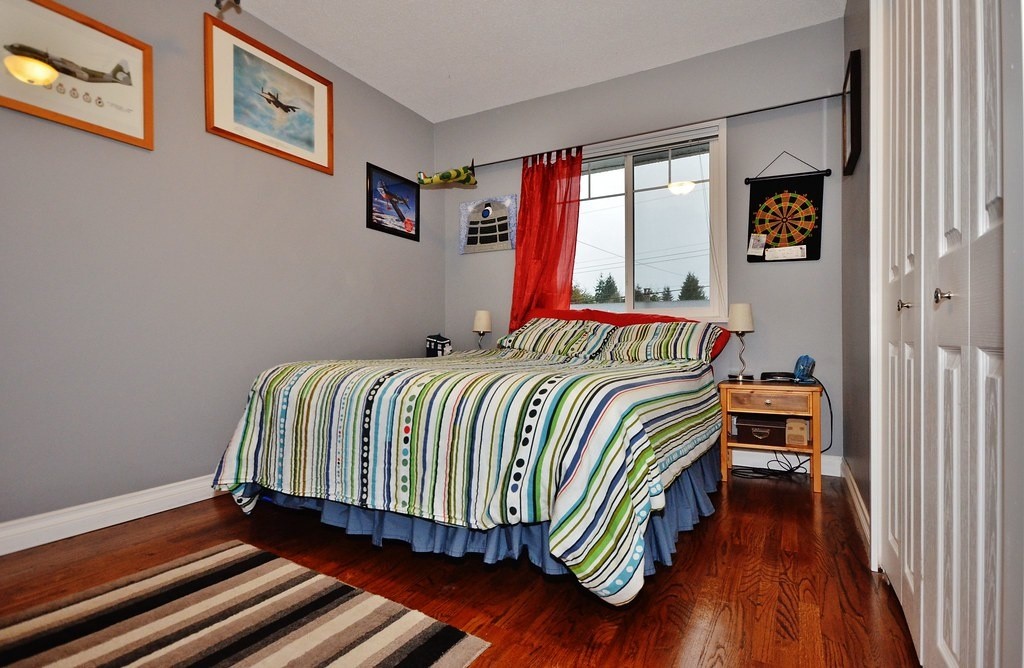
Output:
[718,378,823,494]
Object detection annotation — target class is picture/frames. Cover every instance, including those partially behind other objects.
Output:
[842,49,862,175]
[0,0,156,152]
[204,12,334,176]
[366,162,420,243]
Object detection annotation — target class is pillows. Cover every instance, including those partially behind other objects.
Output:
[604,321,722,366]
[496,317,619,361]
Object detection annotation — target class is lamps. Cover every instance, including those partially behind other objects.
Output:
[726,303,755,382]
[472,310,492,350]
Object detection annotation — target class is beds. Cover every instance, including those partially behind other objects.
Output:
[208,345,724,605]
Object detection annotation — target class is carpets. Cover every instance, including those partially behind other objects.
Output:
[0,537,490,668]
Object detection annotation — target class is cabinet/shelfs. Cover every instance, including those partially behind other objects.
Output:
[871,2,1024,667]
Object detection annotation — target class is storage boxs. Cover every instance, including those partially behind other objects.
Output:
[735,416,787,448]
[786,419,808,447]
[426,334,451,357]
